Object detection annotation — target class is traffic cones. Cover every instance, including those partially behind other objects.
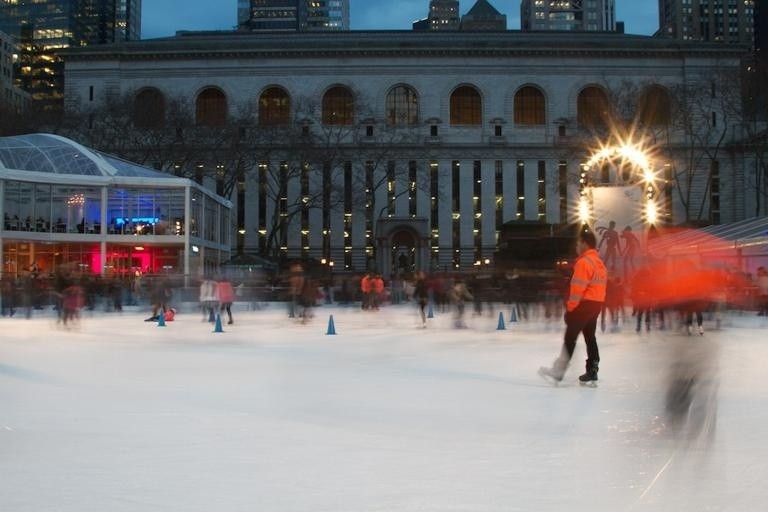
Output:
[157,307,167,326]
[496,312,505,330]
[426,305,434,318]
[208,308,216,322]
[511,307,518,321]
[325,315,338,335]
[213,313,224,332]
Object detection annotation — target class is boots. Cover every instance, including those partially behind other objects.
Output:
[635,320,704,334]
[579,360,598,381]
[539,359,569,381]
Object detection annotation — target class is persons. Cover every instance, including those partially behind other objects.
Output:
[391,272,402,304]
[369,273,383,311]
[435,276,449,313]
[619,224,641,274]
[593,220,623,270]
[535,232,608,382]
[286,264,304,320]
[359,272,374,310]
[4,213,129,234]
[323,282,331,305]
[450,277,475,320]
[340,280,349,303]
[600,265,768,337]
[299,278,316,324]
[413,271,428,328]
[0,261,238,328]
